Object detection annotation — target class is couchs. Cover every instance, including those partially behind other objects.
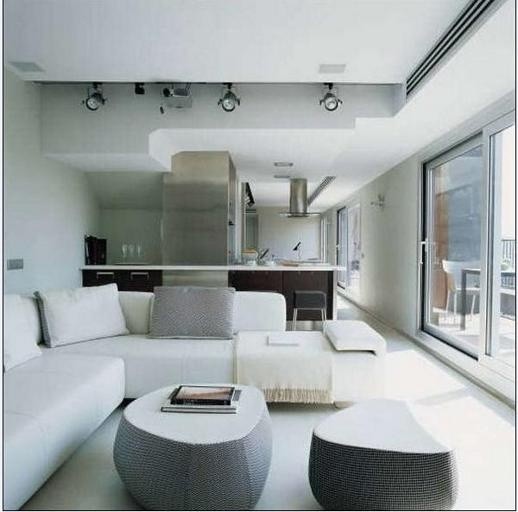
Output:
[3,282,387,509]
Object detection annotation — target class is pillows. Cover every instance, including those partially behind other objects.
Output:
[34,282,131,347]
[146,286,238,340]
[2,294,42,371]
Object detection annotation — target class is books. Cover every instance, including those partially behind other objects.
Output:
[171,385,236,404]
[160,388,242,414]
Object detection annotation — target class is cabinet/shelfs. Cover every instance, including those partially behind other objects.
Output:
[84,271,162,291]
[228,271,333,321]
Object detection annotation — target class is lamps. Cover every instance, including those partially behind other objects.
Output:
[79,84,342,113]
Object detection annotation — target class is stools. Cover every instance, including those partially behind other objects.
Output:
[290,288,327,333]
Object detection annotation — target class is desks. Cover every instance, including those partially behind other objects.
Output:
[459,267,515,334]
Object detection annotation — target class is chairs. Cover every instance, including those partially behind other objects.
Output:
[441,258,485,328]
